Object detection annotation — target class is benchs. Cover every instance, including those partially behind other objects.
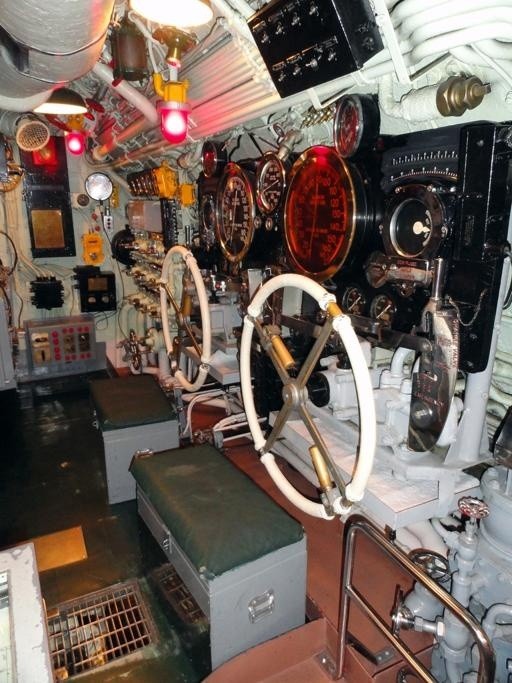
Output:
[89,375,180,505]
[128,443,308,673]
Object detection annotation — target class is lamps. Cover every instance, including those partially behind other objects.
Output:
[85,172,114,214]
[128,0,214,27]
[33,88,88,114]
